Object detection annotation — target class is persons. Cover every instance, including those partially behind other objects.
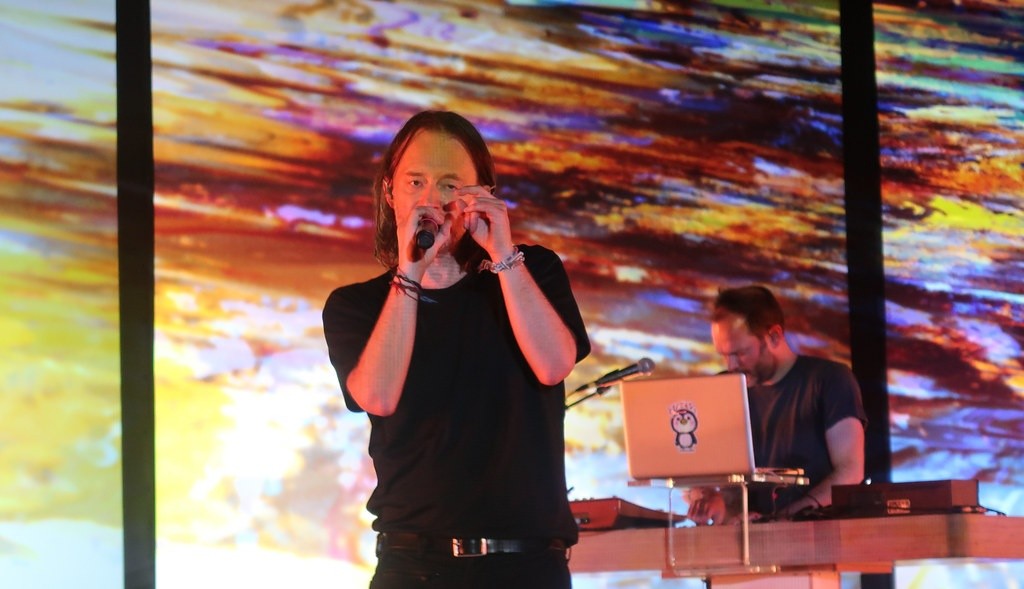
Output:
[686,285,864,524]
[323,111,592,589]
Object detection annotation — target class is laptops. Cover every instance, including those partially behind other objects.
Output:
[620,372,804,478]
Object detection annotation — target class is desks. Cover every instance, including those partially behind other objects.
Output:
[566,514,1024,589]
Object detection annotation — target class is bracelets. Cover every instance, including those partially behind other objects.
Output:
[806,494,823,507]
[390,274,422,294]
[489,246,525,274]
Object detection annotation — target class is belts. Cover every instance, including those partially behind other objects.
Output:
[377,531,566,558]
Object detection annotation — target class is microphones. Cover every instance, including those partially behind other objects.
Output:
[415,217,439,249]
[576,359,655,392]
